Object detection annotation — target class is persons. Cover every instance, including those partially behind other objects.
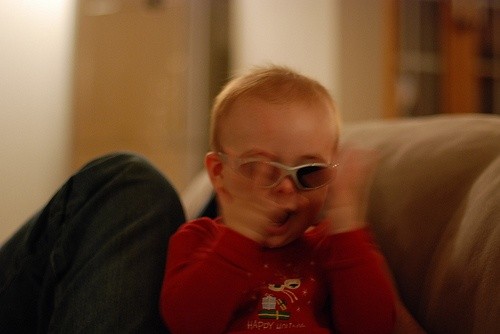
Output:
[158,66,429,334]
[0,152,219,334]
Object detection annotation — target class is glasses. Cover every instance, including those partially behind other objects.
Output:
[216,152,341,191]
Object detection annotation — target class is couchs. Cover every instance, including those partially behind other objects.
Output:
[181,109,500,332]
